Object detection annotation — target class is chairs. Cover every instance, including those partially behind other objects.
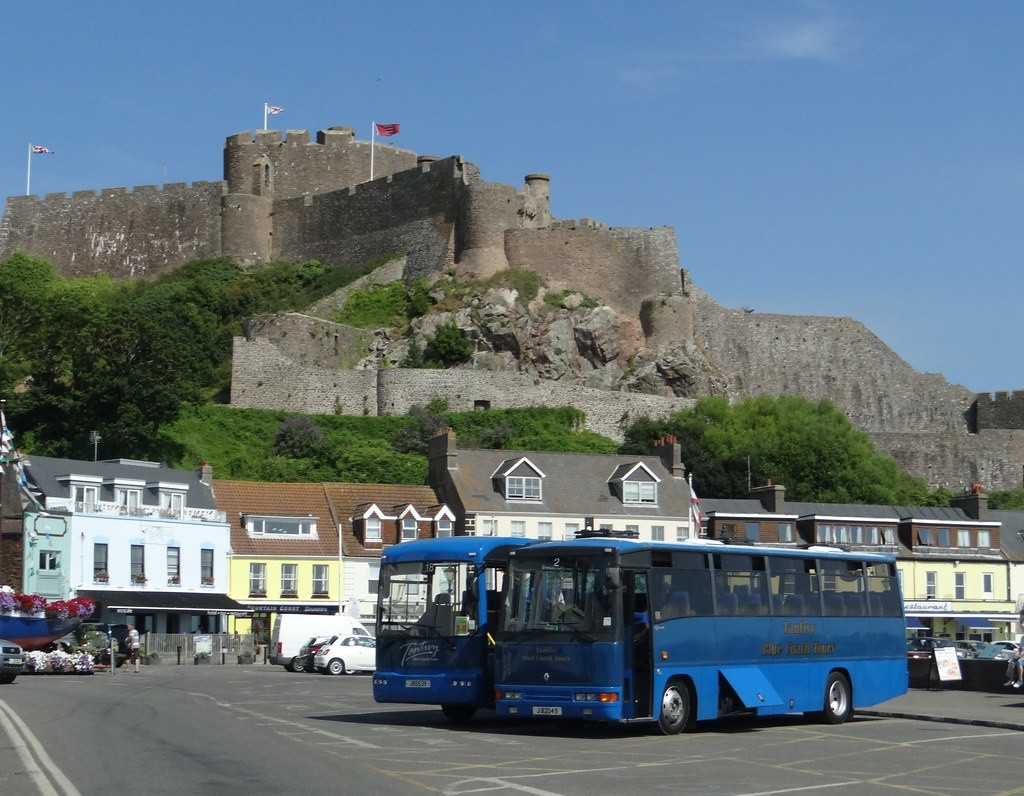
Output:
[659,582,902,620]
[584,590,604,621]
[434,592,452,621]
[461,587,649,627]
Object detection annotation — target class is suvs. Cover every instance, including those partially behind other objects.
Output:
[51,622,131,668]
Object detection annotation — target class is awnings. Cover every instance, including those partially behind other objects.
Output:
[78,590,256,616]
[906,617,930,630]
[956,618,1000,630]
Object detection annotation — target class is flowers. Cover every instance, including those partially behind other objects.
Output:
[0,589,95,624]
[22,648,95,672]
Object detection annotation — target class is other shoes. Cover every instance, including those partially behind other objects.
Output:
[134,670,139,673]
[1004,680,1015,685]
[123,670,129,672]
[1013,680,1023,687]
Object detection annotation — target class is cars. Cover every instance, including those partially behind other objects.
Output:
[295,633,357,674]
[908,636,1021,662]
[0,638,26,684]
[313,634,377,676]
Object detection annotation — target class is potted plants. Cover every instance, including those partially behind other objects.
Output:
[238,651,254,665]
[133,573,148,584]
[193,652,211,666]
[168,576,180,584]
[202,576,215,585]
[78,625,95,644]
[97,569,109,582]
[146,652,161,665]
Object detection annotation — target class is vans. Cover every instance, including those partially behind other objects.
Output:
[268,614,373,673]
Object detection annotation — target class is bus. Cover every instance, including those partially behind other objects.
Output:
[372,535,647,722]
[466,527,909,736]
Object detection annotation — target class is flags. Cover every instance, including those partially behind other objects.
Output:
[375,123,400,137]
[32,145,54,154]
[690,487,701,528]
[267,105,283,115]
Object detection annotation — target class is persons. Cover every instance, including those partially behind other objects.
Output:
[122,624,140,673]
[1004,623,1024,687]
